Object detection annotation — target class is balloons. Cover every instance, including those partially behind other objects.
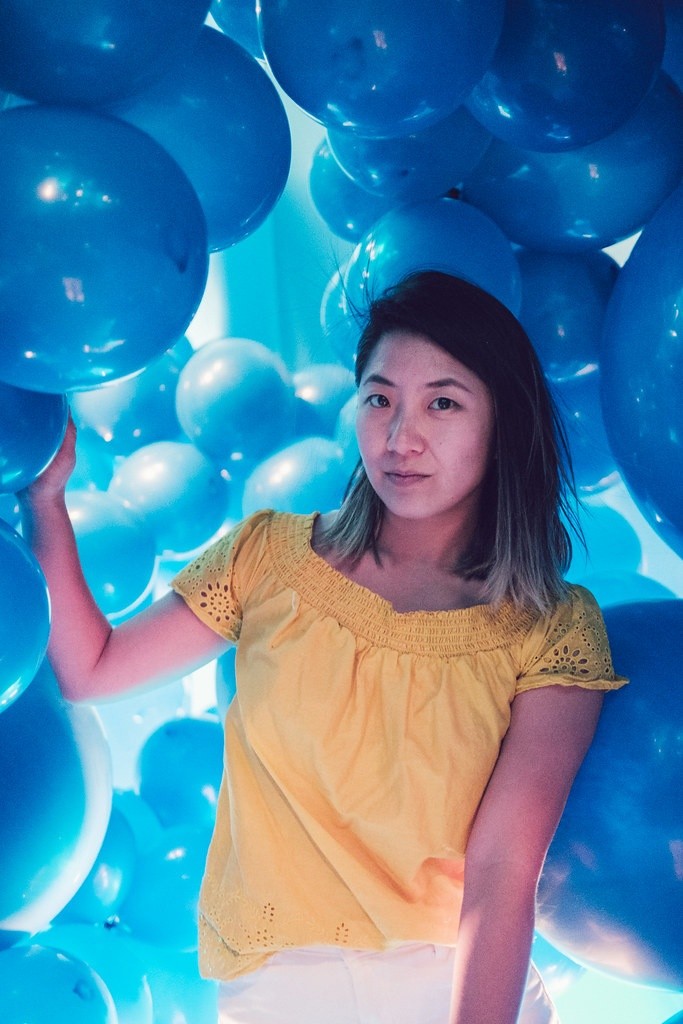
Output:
[0,0,683,1024]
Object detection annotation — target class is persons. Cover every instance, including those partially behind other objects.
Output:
[22,271,627,1024]
